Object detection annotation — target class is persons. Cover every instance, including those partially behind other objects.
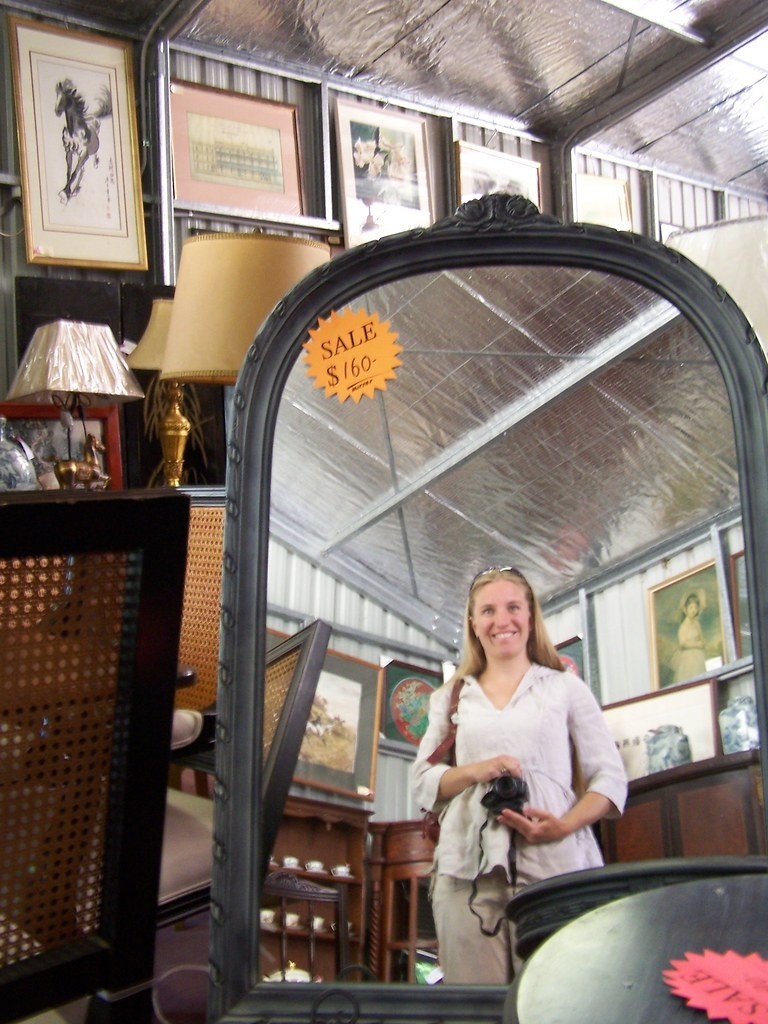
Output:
[405,568,627,983]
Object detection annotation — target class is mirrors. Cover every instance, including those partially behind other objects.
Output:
[204,194,768,1024]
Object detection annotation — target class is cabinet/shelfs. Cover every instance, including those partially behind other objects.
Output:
[598,749,768,864]
[258,794,376,983]
[366,819,440,983]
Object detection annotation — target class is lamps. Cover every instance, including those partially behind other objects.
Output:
[126,298,191,487]
[5,317,146,461]
[159,234,331,385]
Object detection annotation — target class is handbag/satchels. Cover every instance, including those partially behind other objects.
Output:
[422,680,464,844]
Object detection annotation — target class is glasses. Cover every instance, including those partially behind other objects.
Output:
[468,564,522,589]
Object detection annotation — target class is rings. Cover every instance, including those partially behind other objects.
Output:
[500,767,508,773]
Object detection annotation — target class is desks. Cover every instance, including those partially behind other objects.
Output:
[505,854,768,961]
[502,873,768,1024]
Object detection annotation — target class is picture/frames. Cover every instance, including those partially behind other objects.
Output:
[265,627,383,802]
[333,98,437,250]
[646,559,729,693]
[8,15,148,271]
[553,634,584,681]
[575,173,632,233]
[170,78,306,215]
[0,404,124,491]
[601,679,722,781]
[455,139,543,215]
[379,654,443,756]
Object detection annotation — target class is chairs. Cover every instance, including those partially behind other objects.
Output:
[260,620,333,885]
[259,870,350,982]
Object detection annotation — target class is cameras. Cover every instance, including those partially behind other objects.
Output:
[480,774,531,816]
